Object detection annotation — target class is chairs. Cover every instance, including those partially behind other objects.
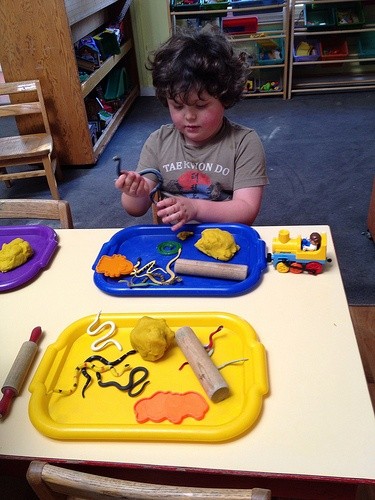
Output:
[1,199,74,229]
[0,225,375,484]
[0,80,67,200]
[26,461,272,500]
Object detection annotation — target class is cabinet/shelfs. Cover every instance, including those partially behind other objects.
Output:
[0,0,141,168]
[166,0,289,101]
[288,0,375,100]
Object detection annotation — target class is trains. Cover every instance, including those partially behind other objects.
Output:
[271,230,333,276]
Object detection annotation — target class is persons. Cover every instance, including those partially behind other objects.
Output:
[114,30,270,231]
[303,232,321,252]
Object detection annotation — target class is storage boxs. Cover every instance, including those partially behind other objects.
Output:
[170,0,284,19]
[293,36,320,68]
[335,2,366,30]
[304,4,336,31]
[243,77,256,93]
[259,69,282,92]
[357,31,375,65]
[320,36,349,66]
[257,39,284,69]
